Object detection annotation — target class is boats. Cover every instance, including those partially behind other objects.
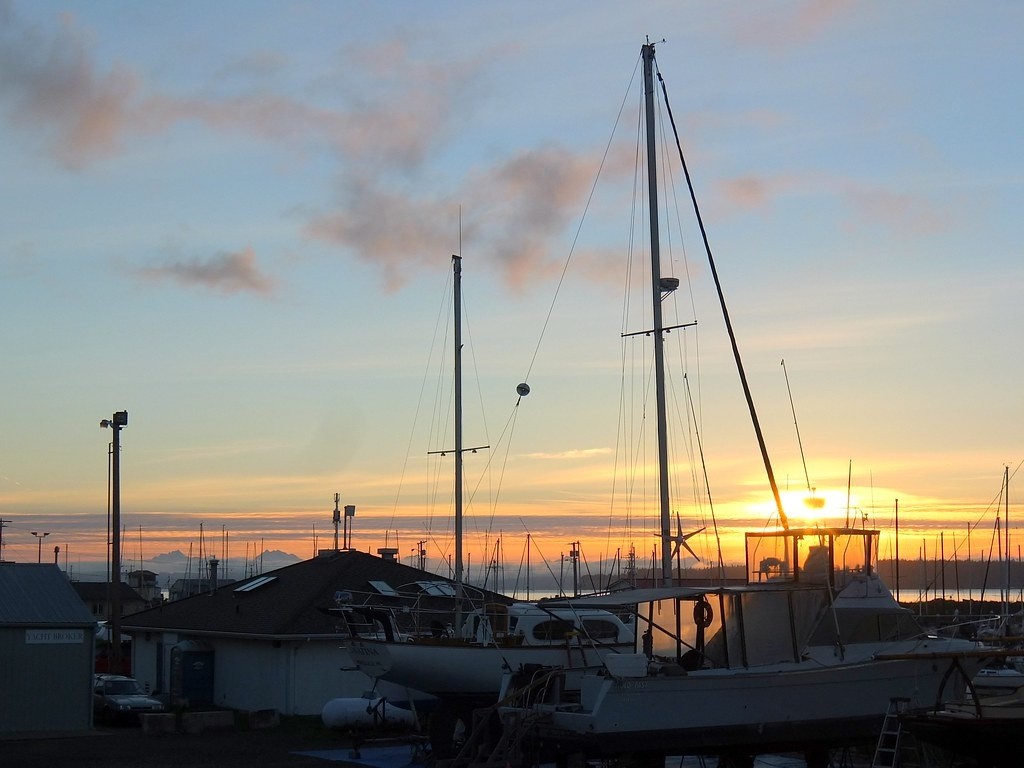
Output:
[496,37,1000,755]
[894,600,1024,767]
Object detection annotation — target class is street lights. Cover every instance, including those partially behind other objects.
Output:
[29,532,49,563]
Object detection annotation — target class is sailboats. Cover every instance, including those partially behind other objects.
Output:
[314,253,636,716]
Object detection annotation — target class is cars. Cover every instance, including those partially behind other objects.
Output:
[93,674,165,726]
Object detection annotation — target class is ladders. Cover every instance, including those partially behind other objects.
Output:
[872,696,929,768]
